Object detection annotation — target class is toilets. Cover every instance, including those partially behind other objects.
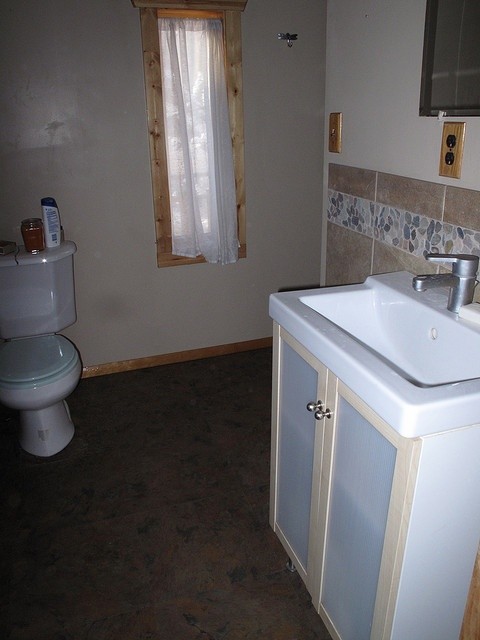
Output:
[0,241,83,456]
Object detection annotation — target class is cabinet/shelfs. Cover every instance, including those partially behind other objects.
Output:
[268,319,480,640]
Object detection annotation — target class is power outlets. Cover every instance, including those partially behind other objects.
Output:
[439,122,466,179]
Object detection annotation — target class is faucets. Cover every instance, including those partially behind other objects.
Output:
[413,252,478,312]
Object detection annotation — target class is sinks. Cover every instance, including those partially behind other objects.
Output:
[299,287,480,388]
